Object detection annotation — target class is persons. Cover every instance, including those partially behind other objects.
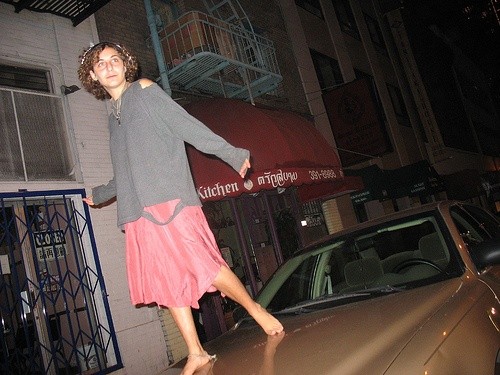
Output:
[78,40,284,375]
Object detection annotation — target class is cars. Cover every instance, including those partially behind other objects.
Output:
[156,199,500,375]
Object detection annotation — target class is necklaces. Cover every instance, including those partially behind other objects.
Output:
[110,87,123,127]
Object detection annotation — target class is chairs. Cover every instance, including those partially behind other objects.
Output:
[331,231,449,295]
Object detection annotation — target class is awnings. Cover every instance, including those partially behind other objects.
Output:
[177,97,346,205]
